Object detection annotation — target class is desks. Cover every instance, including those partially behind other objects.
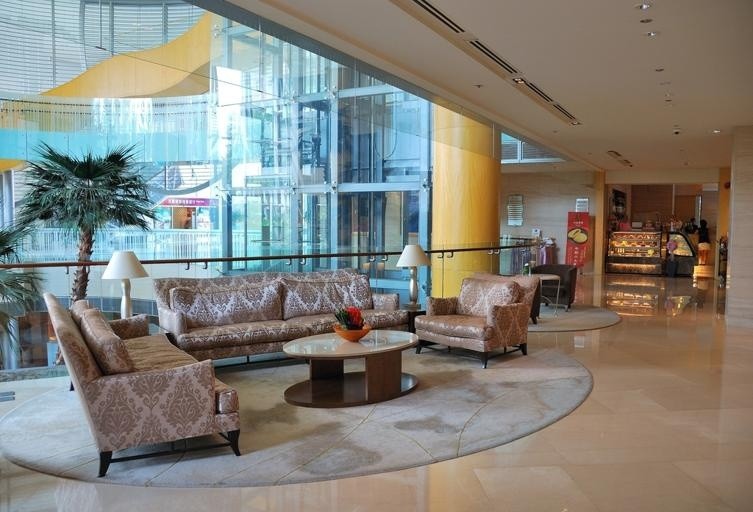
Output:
[535,274,560,320]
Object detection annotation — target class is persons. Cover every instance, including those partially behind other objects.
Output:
[692,278,710,309]
[617,214,632,230]
[697,219,712,265]
[684,217,699,248]
[183,207,193,229]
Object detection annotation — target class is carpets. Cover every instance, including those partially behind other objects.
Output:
[527,304,622,332]
[0,345,594,487]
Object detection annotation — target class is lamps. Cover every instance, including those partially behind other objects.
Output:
[396,244,440,346]
[101,251,150,319]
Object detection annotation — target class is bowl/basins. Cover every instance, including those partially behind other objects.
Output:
[332,325,373,342]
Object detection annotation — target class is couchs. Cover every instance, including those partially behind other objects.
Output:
[415,272,541,369]
[42,292,241,478]
[497,274,540,324]
[152,272,410,361]
[531,264,577,312]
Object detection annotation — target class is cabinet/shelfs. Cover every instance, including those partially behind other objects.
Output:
[665,232,698,278]
[605,231,667,276]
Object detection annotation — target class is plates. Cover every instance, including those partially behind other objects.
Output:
[570,227,588,243]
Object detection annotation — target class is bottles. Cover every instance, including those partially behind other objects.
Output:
[656,221,683,229]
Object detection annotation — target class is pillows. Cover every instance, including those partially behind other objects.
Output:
[279,275,372,320]
[80,310,133,374]
[72,300,90,324]
[455,278,520,318]
[169,281,282,329]
